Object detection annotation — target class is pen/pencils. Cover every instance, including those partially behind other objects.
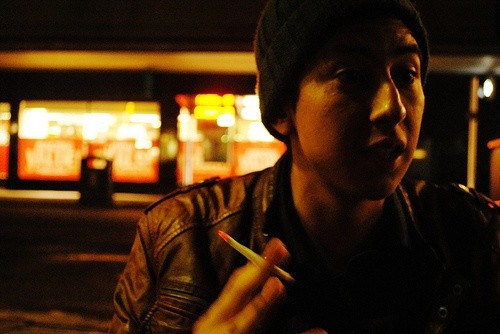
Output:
[219,229,296,283]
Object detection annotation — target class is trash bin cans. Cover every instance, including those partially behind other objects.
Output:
[79,156,114,208]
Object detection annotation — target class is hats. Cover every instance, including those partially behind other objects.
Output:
[256,1,431,143]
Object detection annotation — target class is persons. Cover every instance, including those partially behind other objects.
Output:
[106,1,499,334]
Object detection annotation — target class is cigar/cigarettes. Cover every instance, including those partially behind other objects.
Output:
[217,227,293,283]
